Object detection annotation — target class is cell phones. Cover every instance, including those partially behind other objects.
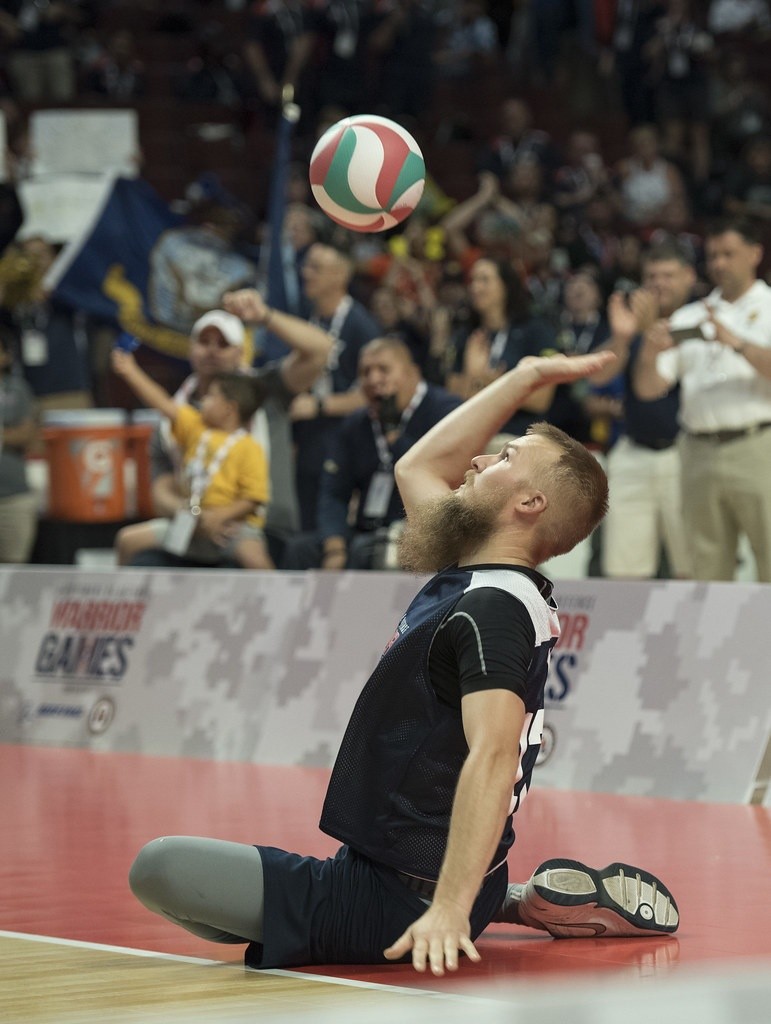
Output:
[669,324,707,344]
[113,333,139,353]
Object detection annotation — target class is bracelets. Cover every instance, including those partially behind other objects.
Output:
[263,306,274,324]
[735,341,746,353]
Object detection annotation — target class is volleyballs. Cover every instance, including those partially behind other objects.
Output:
[306,112,427,235]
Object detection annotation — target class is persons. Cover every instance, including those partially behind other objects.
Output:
[126,345,683,973]
[1,1,770,581]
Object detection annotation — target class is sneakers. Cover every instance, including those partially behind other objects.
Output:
[520,858,679,939]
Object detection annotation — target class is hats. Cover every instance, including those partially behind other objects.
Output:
[193,310,244,348]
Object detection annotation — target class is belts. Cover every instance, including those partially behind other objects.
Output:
[694,422,771,442]
